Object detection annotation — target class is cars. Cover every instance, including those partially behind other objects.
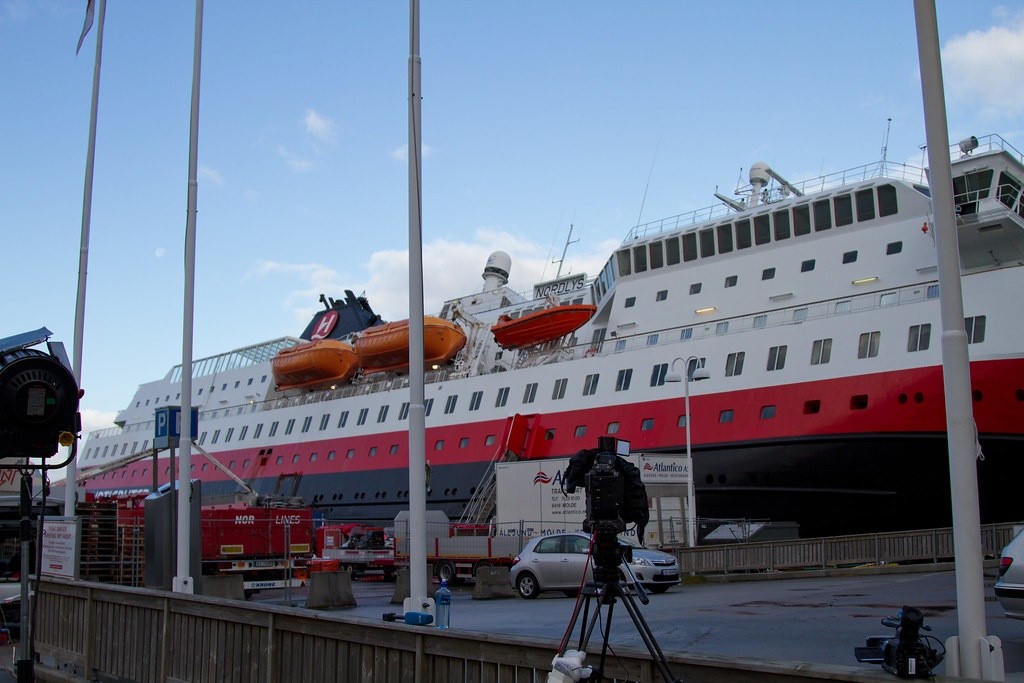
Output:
[994,532,1023,621]
[511,534,683,601]
[0,494,66,609]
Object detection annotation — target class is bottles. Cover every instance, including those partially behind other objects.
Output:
[436,578,451,629]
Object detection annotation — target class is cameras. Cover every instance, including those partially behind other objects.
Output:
[854,605,946,683]
[581,437,650,567]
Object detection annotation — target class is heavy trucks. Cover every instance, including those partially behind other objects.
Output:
[82,453,698,587]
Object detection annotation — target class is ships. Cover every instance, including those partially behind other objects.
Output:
[37,118,1024,540]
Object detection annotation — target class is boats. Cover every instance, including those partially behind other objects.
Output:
[355,315,466,375]
[271,339,355,391]
[490,304,597,352]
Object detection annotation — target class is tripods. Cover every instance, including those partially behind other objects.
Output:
[558,566,680,683]
